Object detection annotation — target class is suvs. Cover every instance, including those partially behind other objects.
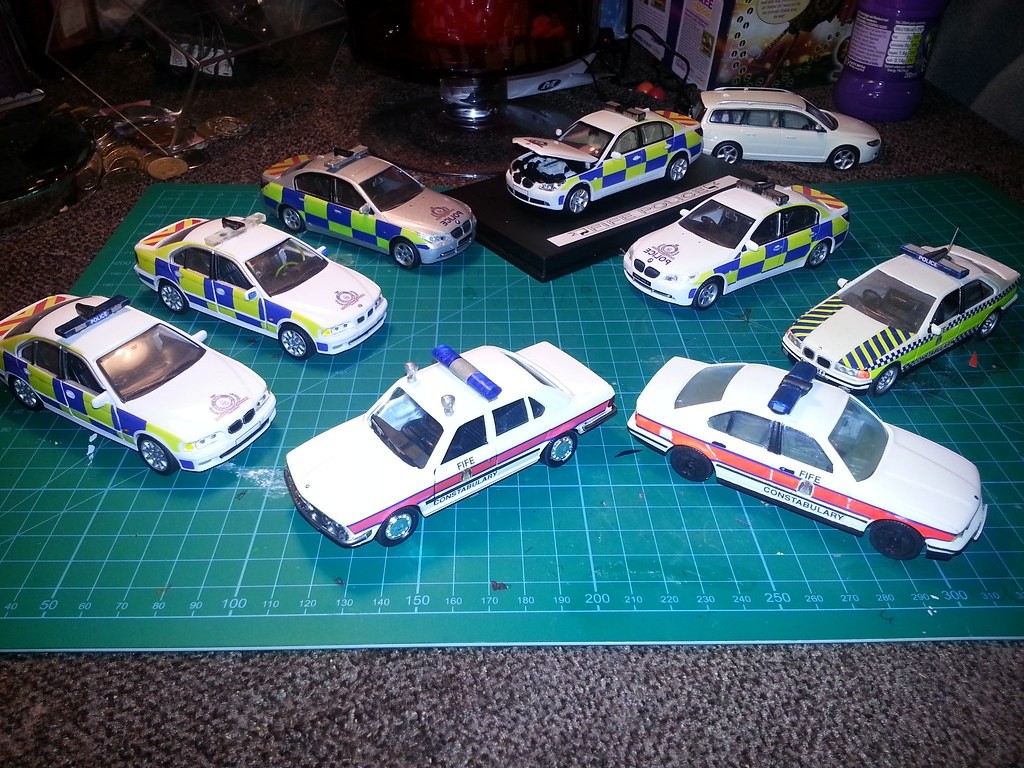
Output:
[696,87,883,172]
[504,103,703,216]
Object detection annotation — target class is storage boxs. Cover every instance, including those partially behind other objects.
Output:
[626,0,857,95]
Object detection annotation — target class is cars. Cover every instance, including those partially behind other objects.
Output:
[137,212,387,355]
[620,180,850,310]
[282,341,619,549]
[782,228,1020,390]
[627,357,990,565]
[0,36,106,209]
[3,285,284,472]
[259,144,479,263]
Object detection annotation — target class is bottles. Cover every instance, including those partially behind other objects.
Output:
[832,0,938,124]
